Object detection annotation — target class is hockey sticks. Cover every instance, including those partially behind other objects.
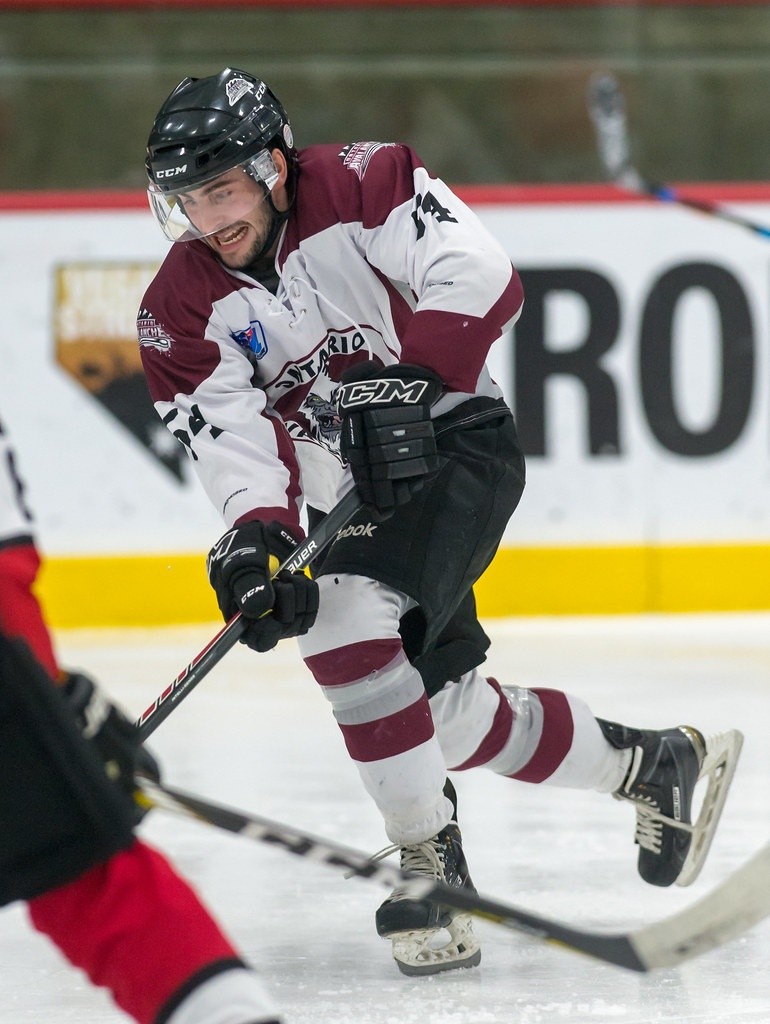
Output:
[590,69,770,240]
[130,481,363,740]
[129,771,770,974]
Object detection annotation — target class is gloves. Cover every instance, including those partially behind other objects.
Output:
[205,521,319,652]
[339,360,445,524]
[64,675,162,827]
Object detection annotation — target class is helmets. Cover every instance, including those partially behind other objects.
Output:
[145,67,297,193]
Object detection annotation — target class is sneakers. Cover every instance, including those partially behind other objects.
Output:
[343,778,481,977]
[595,715,744,888]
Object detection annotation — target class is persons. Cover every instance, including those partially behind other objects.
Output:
[136,68,707,935]
[0,413,282,1024]
